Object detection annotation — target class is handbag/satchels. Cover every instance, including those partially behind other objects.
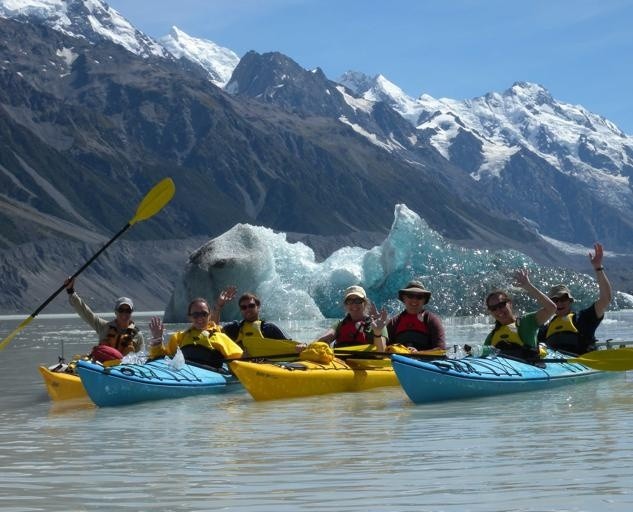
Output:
[93,345,123,361]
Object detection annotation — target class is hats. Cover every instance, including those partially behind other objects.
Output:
[343,286,366,304]
[548,283,575,303]
[114,296,133,311]
[398,280,432,305]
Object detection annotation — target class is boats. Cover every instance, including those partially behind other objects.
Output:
[228,346,450,403]
[388,348,622,406]
[40,356,125,402]
[74,349,249,411]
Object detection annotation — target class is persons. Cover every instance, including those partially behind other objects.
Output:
[211,284,288,354]
[147,297,243,365]
[368,279,446,355]
[314,284,390,362]
[480,265,559,362]
[535,241,612,358]
[63,275,145,361]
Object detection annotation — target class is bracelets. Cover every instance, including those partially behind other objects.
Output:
[372,333,382,338]
[217,303,224,307]
[593,267,604,272]
[212,304,220,312]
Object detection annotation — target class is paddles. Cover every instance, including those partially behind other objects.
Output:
[528,348,633,371]
[0,177,177,350]
[203,344,379,366]
[245,335,448,359]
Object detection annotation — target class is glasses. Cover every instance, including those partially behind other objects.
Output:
[486,300,509,311]
[551,296,570,304]
[189,312,209,318]
[344,298,364,305]
[239,304,257,310]
[116,307,132,313]
[403,293,426,300]
